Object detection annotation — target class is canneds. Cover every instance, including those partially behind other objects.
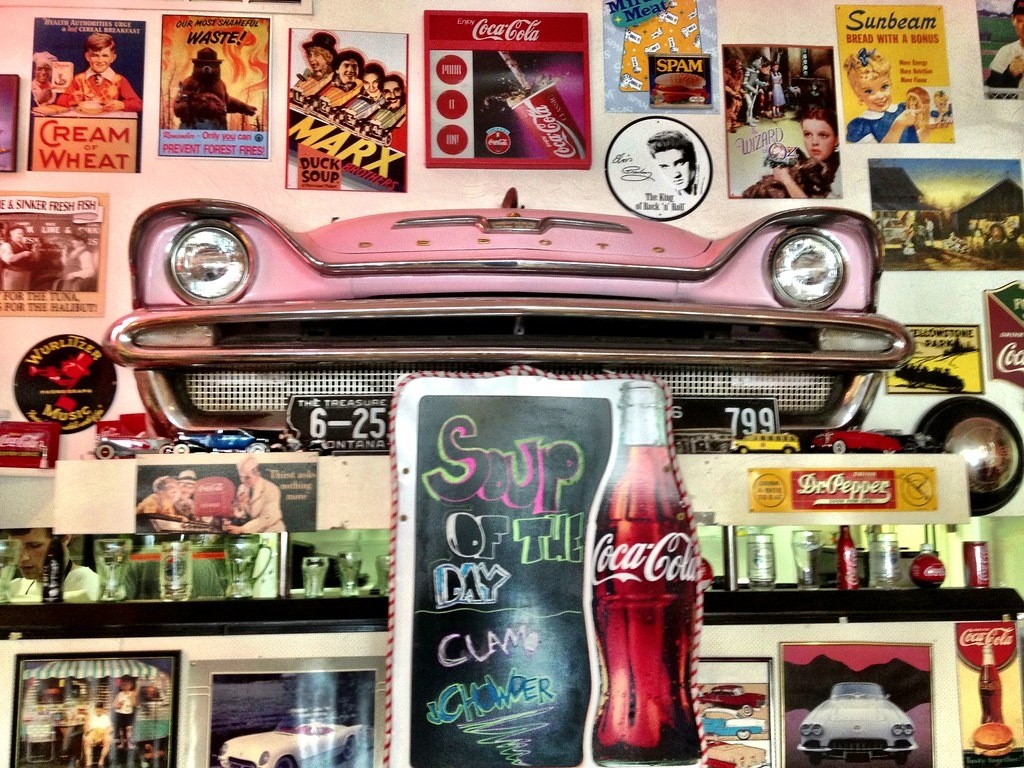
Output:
[962,541,990,589]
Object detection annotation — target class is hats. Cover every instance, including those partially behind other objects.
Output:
[191,48,224,65]
[236,457,259,473]
[5,223,28,237]
[70,228,89,243]
[33,50,59,70]
[301,31,340,57]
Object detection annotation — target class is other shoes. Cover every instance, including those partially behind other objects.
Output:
[774,113,783,118]
[749,118,760,128]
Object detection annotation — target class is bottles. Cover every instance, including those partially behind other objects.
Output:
[837,526,859,590]
[593,383,701,766]
[978,645,1003,726]
[909,544,945,588]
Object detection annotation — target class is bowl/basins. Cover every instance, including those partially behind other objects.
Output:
[80,101,103,113]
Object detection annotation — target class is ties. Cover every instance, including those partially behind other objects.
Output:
[248,488,253,500]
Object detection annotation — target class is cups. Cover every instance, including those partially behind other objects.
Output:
[160,542,192,602]
[871,532,902,589]
[376,555,390,596]
[302,557,328,597]
[511,82,585,161]
[746,534,775,590]
[95,539,132,602]
[224,534,272,598]
[792,530,823,590]
[339,553,361,595]
[0,539,21,604]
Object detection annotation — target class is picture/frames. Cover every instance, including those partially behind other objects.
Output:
[10,649,185,768]
[779,641,937,767]
[186,657,385,768]
[698,656,774,768]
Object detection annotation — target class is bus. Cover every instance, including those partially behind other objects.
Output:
[729,431,803,454]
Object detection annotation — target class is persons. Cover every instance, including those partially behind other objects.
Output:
[33,32,142,111]
[985,0,1024,100]
[0,224,96,291]
[0,528,100,604]
[647,130,709,197]
[303,32,405,110]
[135,454,285,535]
[82,680,137,768]
[724,47,841,199]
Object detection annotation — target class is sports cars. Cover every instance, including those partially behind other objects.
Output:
[815,428,901,454]
[214,708,365,767]
[95,187,916,436]
[172,424,270,454]
[793,682,922,765]
[93,433,173,459]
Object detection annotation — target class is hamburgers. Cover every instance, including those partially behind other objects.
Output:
[651,72,709,105]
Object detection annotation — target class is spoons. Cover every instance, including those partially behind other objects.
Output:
[72,92,93,98]
